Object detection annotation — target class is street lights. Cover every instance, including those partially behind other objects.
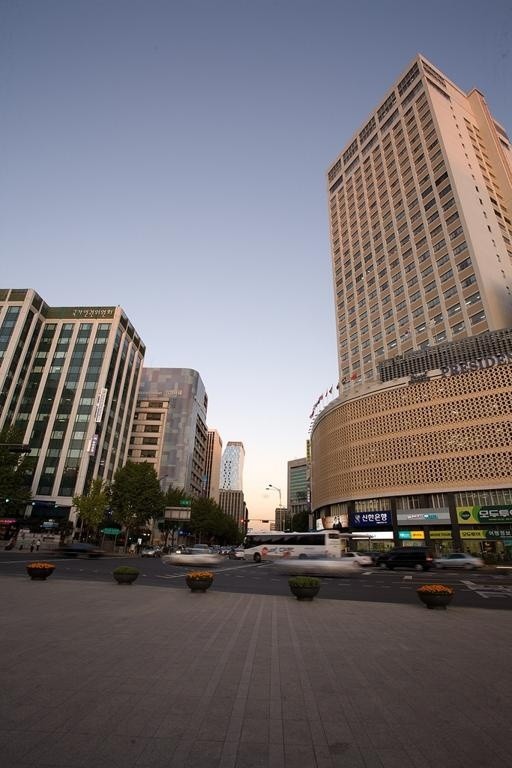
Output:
[265,485,282,531]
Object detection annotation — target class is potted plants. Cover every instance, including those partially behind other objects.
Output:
[286,576,322,602]
[113,566,141,585]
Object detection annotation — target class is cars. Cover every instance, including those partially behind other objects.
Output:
[272,553,363,579]
[53,542,106,558]
[434,551,486,573]
[161,547,222,567]
[342,550,373,566]
[142,544,161,559]
[163,542,243,559]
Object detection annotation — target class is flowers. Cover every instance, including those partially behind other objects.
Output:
[419,586,454,591]
[185,571,214,578]
[29,562,55,568]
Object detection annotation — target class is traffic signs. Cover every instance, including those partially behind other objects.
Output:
[102,528,121,535]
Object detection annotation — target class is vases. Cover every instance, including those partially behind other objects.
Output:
[187,578,214,595]
[420,593,451,611]
[27,568,55,582]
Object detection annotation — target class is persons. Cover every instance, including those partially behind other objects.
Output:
[127,542,140,557]
[0,531,104,552]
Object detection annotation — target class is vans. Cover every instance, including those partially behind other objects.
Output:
[376,545,435,571]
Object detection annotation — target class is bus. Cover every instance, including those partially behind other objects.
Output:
[241,528,342,563]
